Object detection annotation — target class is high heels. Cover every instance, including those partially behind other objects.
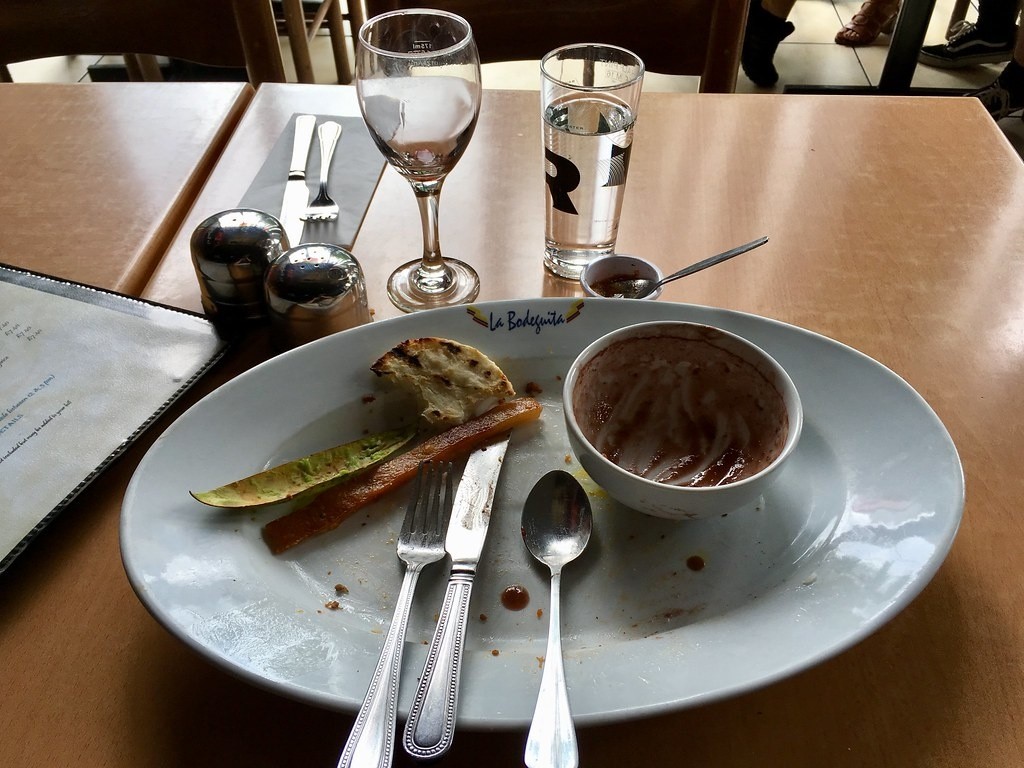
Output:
[835,0,902,46]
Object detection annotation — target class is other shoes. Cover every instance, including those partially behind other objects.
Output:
[741,0,795,88]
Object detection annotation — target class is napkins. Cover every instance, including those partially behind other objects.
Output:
[238,113,387,253]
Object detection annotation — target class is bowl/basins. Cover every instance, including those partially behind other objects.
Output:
[563,320,804,520]
[580,254,664,299]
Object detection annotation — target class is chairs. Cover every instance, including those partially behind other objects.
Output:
[0,0,287,84]
[364,1,754,93]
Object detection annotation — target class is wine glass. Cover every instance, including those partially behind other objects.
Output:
[356,8,482,313]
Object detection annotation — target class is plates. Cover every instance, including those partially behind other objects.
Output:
[119,297,965,726]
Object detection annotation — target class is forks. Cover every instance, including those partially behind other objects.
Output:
[299,121,343,222]
[337,460,452,768]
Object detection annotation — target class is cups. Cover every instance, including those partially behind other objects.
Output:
[540,43,644,280]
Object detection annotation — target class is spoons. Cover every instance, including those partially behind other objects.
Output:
[521,470,593,768]
[610,236,769,296]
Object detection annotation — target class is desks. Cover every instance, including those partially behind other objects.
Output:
[0,83,1024,768]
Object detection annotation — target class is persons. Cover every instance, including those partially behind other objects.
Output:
[917,0,1024,121]
[835,0,903,47]
[741,0,796,87]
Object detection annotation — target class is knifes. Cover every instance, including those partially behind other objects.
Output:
[279,115,316,250]
[403,428,512,758]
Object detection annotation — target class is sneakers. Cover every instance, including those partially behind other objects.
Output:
[916,20,1018,69]
[963,77,1024,121]
